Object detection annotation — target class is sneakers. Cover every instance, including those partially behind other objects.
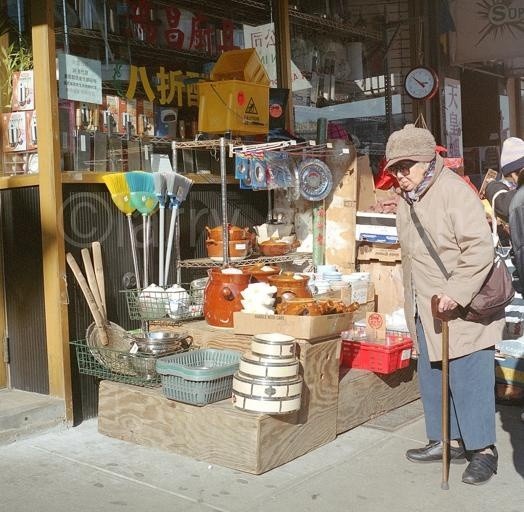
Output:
[462,446,498,485]
[406,440,466,464]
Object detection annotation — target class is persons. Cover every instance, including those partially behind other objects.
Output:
[383,123,506,486]
[485,136,524,247]
[507,167,524,288]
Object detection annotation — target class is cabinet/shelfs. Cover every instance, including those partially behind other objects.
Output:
[16,0,393,171]
[170,138,326,292]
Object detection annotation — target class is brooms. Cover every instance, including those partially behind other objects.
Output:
[101,170,195,330]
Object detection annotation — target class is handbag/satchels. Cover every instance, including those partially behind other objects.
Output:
[461,189,524,323]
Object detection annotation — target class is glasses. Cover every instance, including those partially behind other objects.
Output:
[388,163,417,175]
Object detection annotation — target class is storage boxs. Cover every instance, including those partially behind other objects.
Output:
[195,81,271,142]
[210,47,271,87]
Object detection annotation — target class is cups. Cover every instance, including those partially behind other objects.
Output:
[5,154,39,176]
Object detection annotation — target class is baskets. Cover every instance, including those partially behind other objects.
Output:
[69,285,242,407]
[340,326,418,374]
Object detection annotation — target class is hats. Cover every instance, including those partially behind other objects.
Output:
[384,124,436,170]
[501,137,524,177]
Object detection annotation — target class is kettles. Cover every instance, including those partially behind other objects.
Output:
[81,104,150,132]
[7,83,37,148]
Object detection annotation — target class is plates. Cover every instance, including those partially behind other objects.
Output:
[297,158,333,202]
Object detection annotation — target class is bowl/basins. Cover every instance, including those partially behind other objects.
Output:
[126,331,192,382]
[138,277,209,321]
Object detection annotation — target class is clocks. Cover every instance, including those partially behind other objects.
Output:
[404,65,439,103]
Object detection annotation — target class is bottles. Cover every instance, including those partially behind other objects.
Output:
[203,221,369,328]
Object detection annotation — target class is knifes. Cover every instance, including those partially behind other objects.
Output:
[76,108,153,172]
